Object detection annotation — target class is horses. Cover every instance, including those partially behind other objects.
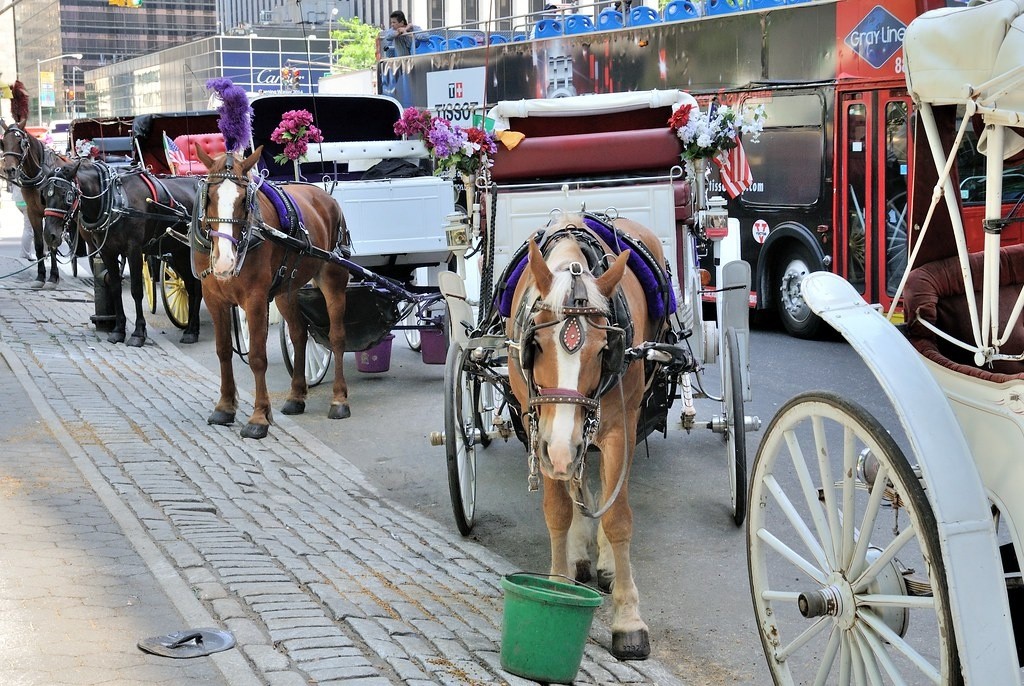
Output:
[194,141,352,440]
[0,119,74,290]
[505,216,673,662]
[41,161,205,348]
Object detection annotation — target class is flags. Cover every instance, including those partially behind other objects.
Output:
[166,136,187,165]
[708,103,754,201]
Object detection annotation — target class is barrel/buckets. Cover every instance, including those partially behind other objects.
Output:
[499,571,604,684]
[419,329,445,365]
[355,333,395,373]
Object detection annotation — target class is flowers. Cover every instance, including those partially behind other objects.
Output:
[666,102,768,162]
[270,108,325,166]
[75,139,100,159]
[392,106,498,177]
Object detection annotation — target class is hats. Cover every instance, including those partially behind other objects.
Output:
[544,3,557,10]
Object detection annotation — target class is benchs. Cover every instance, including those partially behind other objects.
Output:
[901,242,1024,383]
[173,133,227,174]
[92,136,132,165]
[486,89,700,180]
[244,93,406,183]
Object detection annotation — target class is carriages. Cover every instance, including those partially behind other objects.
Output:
[431,90,761,661]
[0,95,455,439]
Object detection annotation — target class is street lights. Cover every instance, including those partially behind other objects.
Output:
[308,35,317,95]
[329,8,338,74]
[37,54,83,127]
[250,33,257,98]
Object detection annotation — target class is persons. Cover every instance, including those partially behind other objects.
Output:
[385,11,429,57]
[11,185,49,261]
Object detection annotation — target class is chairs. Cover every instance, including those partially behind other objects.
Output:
[386,0,812,58]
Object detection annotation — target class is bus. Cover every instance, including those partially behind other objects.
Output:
[371,0,1024,338]
